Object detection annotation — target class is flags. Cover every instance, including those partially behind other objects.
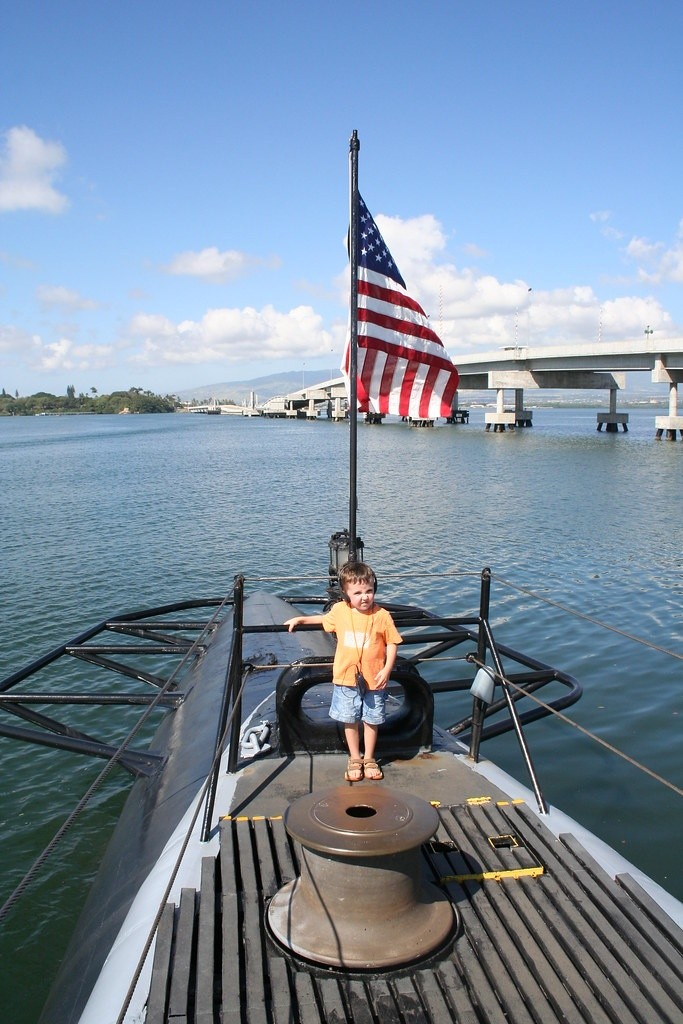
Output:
[340,192,460,418]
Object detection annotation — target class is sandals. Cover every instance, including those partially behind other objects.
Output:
[344,757,363,782]
[363,757,383,780]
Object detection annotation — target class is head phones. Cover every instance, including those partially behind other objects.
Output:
[338,563,377,603]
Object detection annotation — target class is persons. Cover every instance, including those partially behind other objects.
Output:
[284,561,404,781]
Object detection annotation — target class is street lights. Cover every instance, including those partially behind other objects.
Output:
[302,362,305,389]
[329,349,333,381]
[526,287,533,348]
[644,325,653,340]
